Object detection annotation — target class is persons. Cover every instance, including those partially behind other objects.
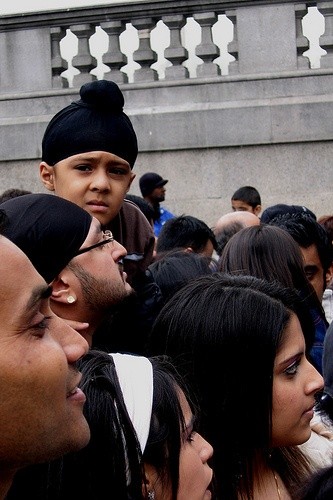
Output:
[38,79,156,339]
[0,233,93,500]
[150,271,333,500]
[0,174,333,500]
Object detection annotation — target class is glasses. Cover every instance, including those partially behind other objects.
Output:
[74,230,114,255]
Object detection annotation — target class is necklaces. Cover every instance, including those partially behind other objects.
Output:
[272,468,282,500]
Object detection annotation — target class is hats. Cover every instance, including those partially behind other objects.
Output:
[139,172,168,198]
[41,80,139,170]
[0,193,92,284]
